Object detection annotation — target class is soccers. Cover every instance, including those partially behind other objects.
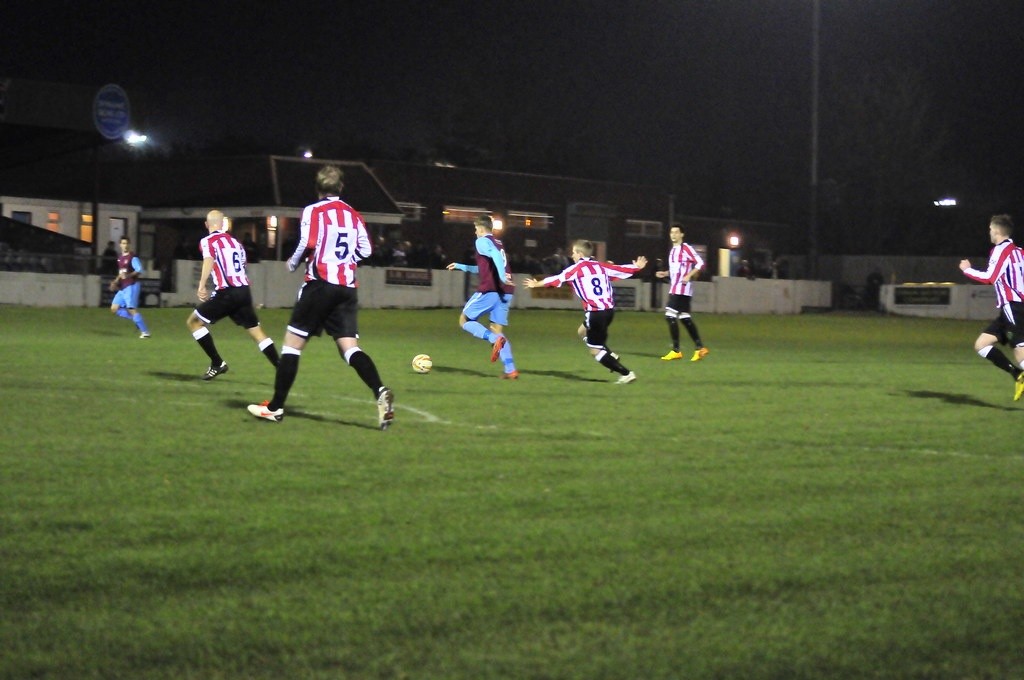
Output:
[412,353,433,374]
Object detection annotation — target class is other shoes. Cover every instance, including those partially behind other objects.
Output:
[614,371,636,384]
[139,332,150,339]
[607,352,620,373]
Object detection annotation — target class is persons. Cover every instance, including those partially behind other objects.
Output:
[366,235,576,275]
[656,224,709,362]
[446,214,519,380]
[108,234,153,340]
[185,208,281,382]
[521,239,648,386]
[246,165,394,431]
[958,213,1024,402]
[153,231,270,292]
[101,240,118,274]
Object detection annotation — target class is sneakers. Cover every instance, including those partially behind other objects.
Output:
[247,400,283,422]
[201,361,228,381]
[376,387,394,430]
[490,336,506,362]
[660,350,682,361]
[691,347,710,362]
[499,370,518,379]
[1014,371,1024,401]
